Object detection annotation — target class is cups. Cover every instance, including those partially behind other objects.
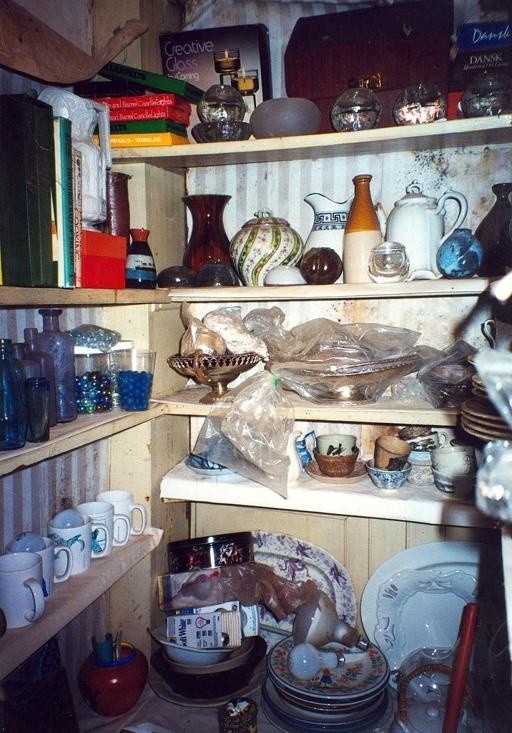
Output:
[46,513,111,578]
[212,48,242,73]
[0,551,46,631]
[483,316,512,353]
[75,501,131,559]
[75,348,157,413]
[5,535,73,600]
[228,67,260,98]
[95,490,148,543]
[407,431,449,452]
[374,436,412,471]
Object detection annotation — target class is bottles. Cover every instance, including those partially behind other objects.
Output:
[341,173,383,283]
[471,181,512,277]
[1,308,75,452]
[124,228,157,289]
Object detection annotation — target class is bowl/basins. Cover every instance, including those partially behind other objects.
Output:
[146,622,234,666]
[364,458,412,489]
[406,449,476,493]
[314,433,357,457]
[399,424,431,439]
[150,636,268,700]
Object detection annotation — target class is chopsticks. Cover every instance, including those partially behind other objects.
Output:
[257,632,394,733]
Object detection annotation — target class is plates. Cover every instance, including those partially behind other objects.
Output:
[459,374,512,443]
[304,455,369,484]
[361,538,482,695]
[254,529,359,657]
[146,652,270,709]
[184,450,237,476]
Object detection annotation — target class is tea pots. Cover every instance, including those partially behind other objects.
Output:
[300,191,388,283]
[375,178,469,280]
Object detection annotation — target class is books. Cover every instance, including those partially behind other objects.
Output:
[1,93,84,289]
